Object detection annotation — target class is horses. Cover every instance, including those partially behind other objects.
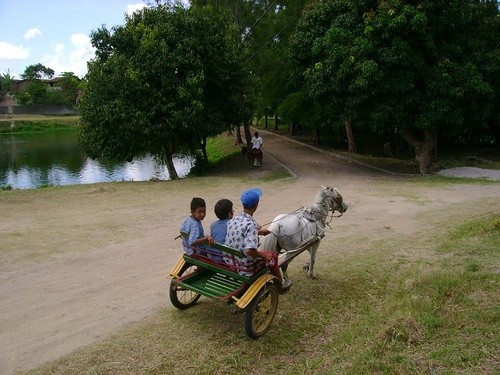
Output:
[241,146,263,170]
[257,185,349,288]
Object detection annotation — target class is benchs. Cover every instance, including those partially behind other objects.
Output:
[180,231,269,284]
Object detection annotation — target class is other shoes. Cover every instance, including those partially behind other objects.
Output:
[282,279,291,288]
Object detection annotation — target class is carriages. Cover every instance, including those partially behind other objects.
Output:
[167,184,348,339]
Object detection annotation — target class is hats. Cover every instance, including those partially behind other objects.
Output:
[240,188,262,207]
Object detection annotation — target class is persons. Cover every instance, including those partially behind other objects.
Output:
[222,188,294,291]
[180,197,216,257]
[206,199,262,262]
[248,132,263,169]
